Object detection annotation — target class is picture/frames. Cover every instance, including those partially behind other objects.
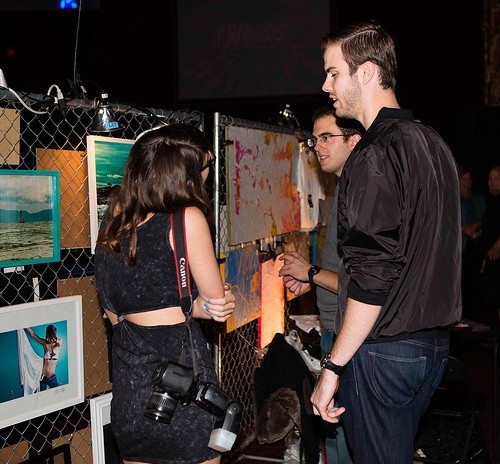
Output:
[86,135,140,254]
[90,392,124,464]
[0,295,84,429]
[0,169,61,268]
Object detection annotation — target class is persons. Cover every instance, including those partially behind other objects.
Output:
[278,109,366,464]
[459,166,500,321]
[310,19,463,464]
[96,123,235,464]
[26,325,63,391]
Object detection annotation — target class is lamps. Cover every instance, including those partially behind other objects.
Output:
[274,103,301,130]
[89,87,125,132]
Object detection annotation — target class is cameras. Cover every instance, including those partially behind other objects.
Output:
[144,360,245,452]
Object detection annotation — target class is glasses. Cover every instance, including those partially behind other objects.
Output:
[307,135,349,147]
[191,152,216,175]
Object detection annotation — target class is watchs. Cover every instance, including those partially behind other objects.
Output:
[320,351,346,376]
[308,265,321,284]
